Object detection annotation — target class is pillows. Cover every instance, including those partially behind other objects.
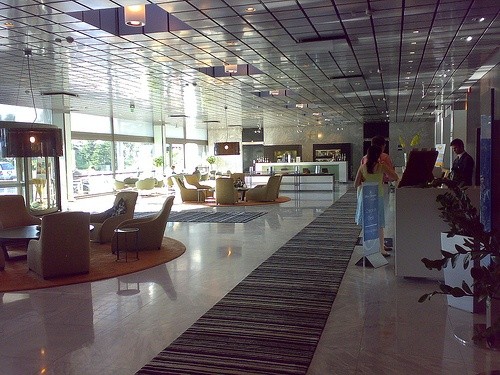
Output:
[111,199,127,216]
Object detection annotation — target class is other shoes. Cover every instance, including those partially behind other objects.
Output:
[381,250,390,257]
[384,245,392,251]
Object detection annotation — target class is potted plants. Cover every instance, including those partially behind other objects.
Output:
[418,168,500,313]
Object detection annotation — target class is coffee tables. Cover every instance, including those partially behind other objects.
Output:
[236,187,249,201]
[0,224,95,271]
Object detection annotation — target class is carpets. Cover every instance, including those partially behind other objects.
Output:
[199,196,291,206]
[0,236,186,291]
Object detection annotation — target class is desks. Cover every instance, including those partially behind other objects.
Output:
[245,174,335,192]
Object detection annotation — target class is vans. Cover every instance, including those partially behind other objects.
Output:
[0,162,17,181]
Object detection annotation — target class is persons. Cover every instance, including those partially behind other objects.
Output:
[170,166,179,174]
[353,144,399,257]
[193,166,200,174]
[359,136,395,251]
[448,139,475,187]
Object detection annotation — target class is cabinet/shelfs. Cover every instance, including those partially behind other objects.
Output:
[255,160,349,184]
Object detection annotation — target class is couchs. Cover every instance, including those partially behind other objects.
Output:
[231,173,246,186]
[0,195,43,228]
[246,173,282,202]
[111,196,175,255]
[215,178,239,205]
[185,175,215,200]
[89,191,138,245]
[115,177,164,190]
[26,212,91,280]
[174,176,205,203]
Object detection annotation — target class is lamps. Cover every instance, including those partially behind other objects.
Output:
[124,0,147,28]
[225,65,240,74]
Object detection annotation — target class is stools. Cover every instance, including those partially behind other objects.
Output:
[113,227,140,263]
[197,188,207,202]
